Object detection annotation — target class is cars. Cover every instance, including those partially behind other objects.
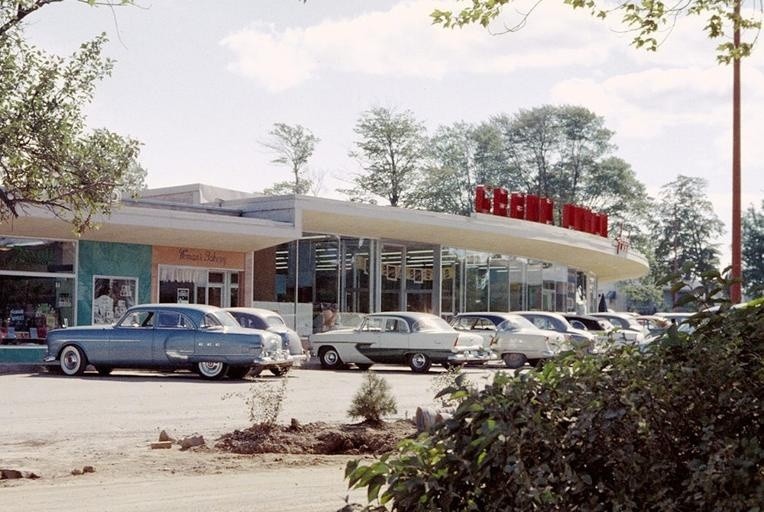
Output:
[45,302,308,381]
[308,311,485,375]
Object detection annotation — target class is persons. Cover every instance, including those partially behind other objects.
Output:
[670,318,677,326]
[141,311,153,326]
[577,294,588,315]
[313,302,339,333]
